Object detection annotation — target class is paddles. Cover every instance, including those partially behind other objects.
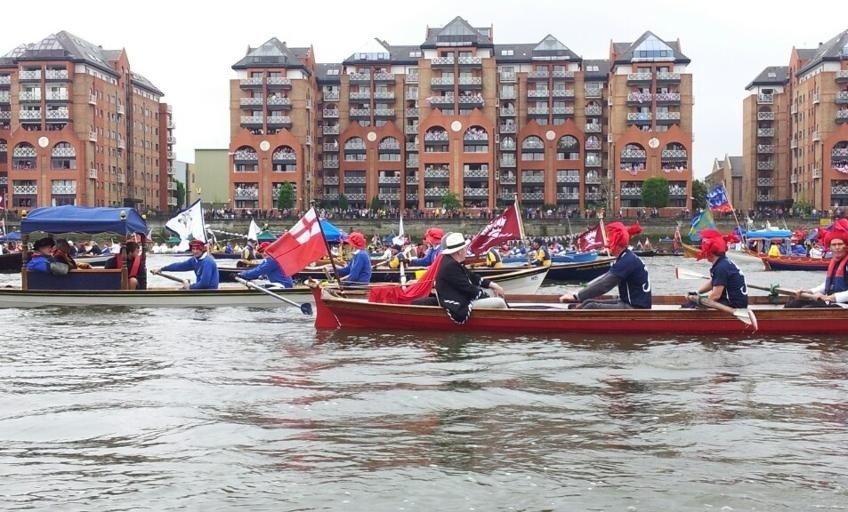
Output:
[232,273,313,316]
[688,293,758,331]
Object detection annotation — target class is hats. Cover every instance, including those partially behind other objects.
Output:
[38,238,55,247]
[391,245,401,250]
[189,240,206,252]
[442,232,473,255]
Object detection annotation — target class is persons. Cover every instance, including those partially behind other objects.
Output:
[149,239,220,289]
[49,239,93,270]
[430,232,508,327]
[315,229,576,271]
[333,233,372,284]
[781,217,848,312]
[766,238,833,260]
[100,242,145,290]
[682,227,749,310]
[21,237,69,275]
[277,204,848,219]
[0,185,285,267]
[237,242,295,287]
[557,220,651,309]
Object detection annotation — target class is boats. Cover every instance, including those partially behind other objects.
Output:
[315,287,848,339]
[2,251,133,266]
[759,249,837,273]
[499,251,597,265]
[212,250,263,259]
[0,269,556,308]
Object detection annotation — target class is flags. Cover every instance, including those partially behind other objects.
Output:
[467,194,526,257]
[704,184,734,216]
